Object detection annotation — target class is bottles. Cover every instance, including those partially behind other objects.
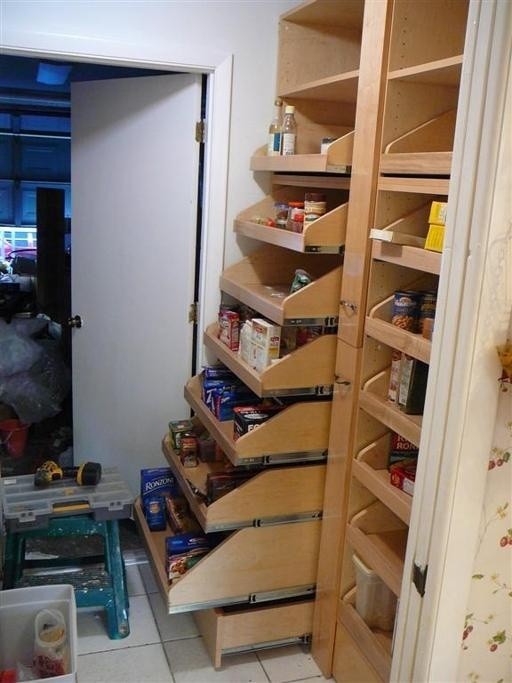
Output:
[267,99,283,157]
[280,105,297,155]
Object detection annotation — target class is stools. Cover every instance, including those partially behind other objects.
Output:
[1,518,131,641]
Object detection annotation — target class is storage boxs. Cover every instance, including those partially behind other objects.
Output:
[0,584,80,683]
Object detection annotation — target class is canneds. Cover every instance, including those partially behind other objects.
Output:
[303,201,326,234]
[391,289,438,334]
[286,201,304,234]
